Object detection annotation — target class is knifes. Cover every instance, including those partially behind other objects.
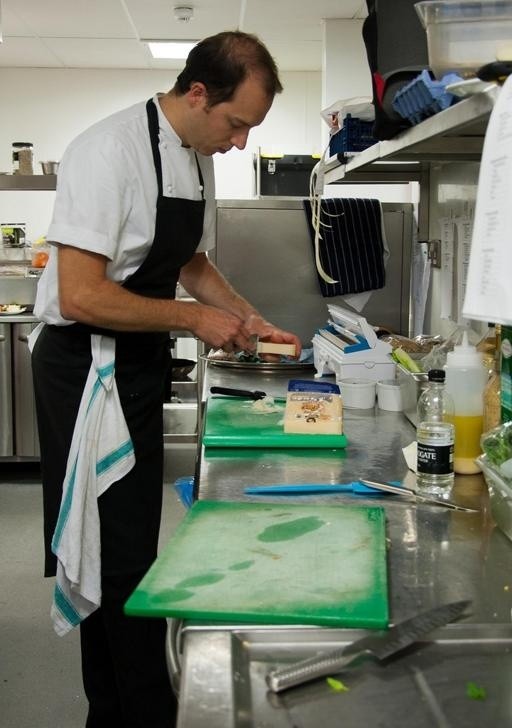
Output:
[210,387,287,402]
[359,477,480,513]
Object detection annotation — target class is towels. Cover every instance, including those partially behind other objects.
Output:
[49,332,138,643]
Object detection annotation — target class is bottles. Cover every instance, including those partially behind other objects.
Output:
[11,142,34,175]
[438,330,490,475]
[415,369,455,499]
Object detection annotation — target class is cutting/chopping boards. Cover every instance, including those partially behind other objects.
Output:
[123,498,390,630]
[200,391,348,450]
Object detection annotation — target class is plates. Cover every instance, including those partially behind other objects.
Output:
[0,310,26,315]
[199,348,314,369]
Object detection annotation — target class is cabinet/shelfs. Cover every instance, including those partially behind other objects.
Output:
[1,324,41,464]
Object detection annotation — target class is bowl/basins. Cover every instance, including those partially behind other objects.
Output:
[336,376,376,409]
[376,379,403,412]
[42,161,60,175]
[171,358,197,379]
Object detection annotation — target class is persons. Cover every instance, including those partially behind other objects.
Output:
[26,29,302,728]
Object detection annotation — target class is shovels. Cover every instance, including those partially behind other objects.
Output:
[243,475,476,511]
